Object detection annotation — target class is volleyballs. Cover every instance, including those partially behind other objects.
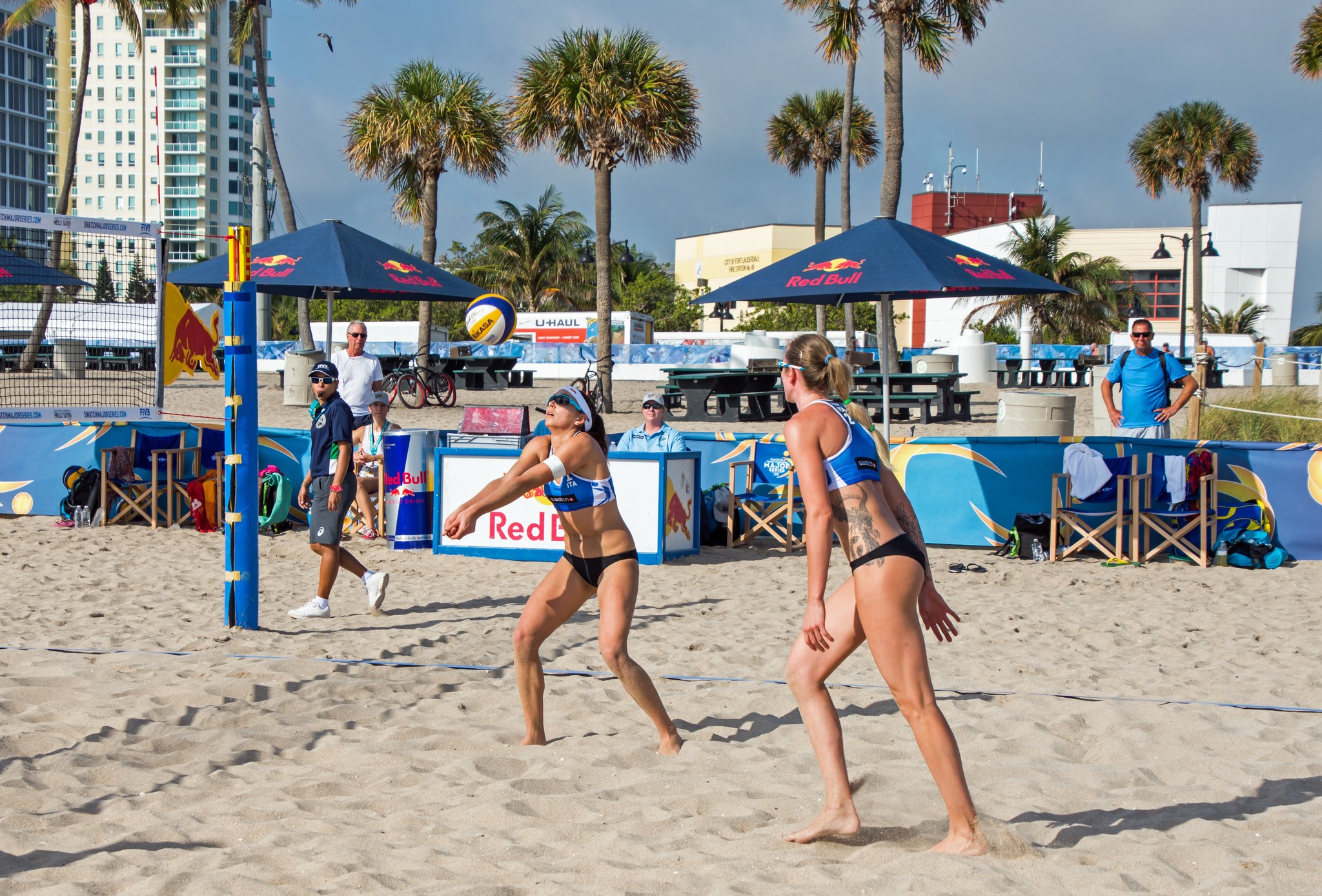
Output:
[116,496,143,521]
[64,465,88,490]
[464,293,517,346]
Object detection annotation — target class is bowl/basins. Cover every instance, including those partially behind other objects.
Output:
[1168,554,1193,564]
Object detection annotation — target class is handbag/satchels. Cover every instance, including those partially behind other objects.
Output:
[994,512,1065,561]
[1212,528,1289,569]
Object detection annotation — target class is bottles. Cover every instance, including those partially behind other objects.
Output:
[307,507,353,533]
[1031,539,1044,563]
[1217,541,1227,567]
[90,506,105,527]
[1042,544,1072,560]
[82,506,90,528]
[74,505,82,528]
[1242,534,1259,546]
[1213,543,1226,565]
[608,442,617,451]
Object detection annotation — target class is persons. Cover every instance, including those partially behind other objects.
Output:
[1091,343,1098,356]
[330,320,384,431]
[352,391,401,542]
[617,393,687,453]
[776,334,991,858]
[1163,342,1174,356]
[1101,318,1199,439]
[441,386,683,759]
[1202,338,1215,370]
[288,362,389,620]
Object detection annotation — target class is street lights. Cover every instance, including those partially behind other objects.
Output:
[1149,230,1222,367]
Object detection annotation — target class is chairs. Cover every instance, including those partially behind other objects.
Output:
[340,442,388,539]
[1046,454,1136,572]
[723,440,806,563]
[99,429,185,527]
[166,427,229,532]
[1126,446,1224,577]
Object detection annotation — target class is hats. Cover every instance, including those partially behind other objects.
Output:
[1163,342,1168,346]
[369,391,389,405]
[1202,338,1208,341]
[308,361,339,378]
[642,392,664,407]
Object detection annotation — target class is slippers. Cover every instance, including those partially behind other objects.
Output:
[50,519,75,527]
[948,563,987,573]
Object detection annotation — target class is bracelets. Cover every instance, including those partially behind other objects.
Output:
[330,490,341,494]
[302,481,310,486]
[300,485,310,488]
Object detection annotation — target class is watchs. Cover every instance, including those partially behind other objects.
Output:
[330,485,343,492]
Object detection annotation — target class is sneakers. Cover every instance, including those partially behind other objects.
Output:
[287,598,331,619]
[359,528,378,541]
[364,571,390,611]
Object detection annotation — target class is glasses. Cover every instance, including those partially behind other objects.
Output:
[347,332,368,338]
[1091,346,1093,348]
[1132,332,1152,338]
[310,377,335,384]
[546,394,590,422]
[643,403,663,409]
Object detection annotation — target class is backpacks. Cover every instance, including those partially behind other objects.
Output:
[59,468,109,522]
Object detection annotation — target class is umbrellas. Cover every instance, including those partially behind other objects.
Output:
[690,215,1081,465]
[142,218,490,362]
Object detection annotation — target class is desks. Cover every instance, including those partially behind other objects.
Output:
[668,371,776,422]
[1040,355,1080,389]
[847,366,969,417]
[0,343,54,368]
[658,367,749,410]
[375,349,415,362]
[450,352,523,367]
[998,353,1038,385]
[86,345,156,372]
[1170,353,1219,387]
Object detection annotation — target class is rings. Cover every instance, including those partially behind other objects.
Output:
[331,505,335,507]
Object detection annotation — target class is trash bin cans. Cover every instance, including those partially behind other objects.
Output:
[52,339,87,379]
[383,427,442,550]
[1272,352,1299,386]
[282,350,327,409]
[331,342,349,353]
[910,354,961,417]
[996,390,1077,437]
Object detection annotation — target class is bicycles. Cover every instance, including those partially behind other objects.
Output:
[569,348,616,414]
[381,340,457,409]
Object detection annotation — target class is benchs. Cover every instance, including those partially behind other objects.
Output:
[990,354,1097,390]
[326,347,539,396]
[1183,368,1229,387]
[653,361,980,427]
[0,350,141,376]
